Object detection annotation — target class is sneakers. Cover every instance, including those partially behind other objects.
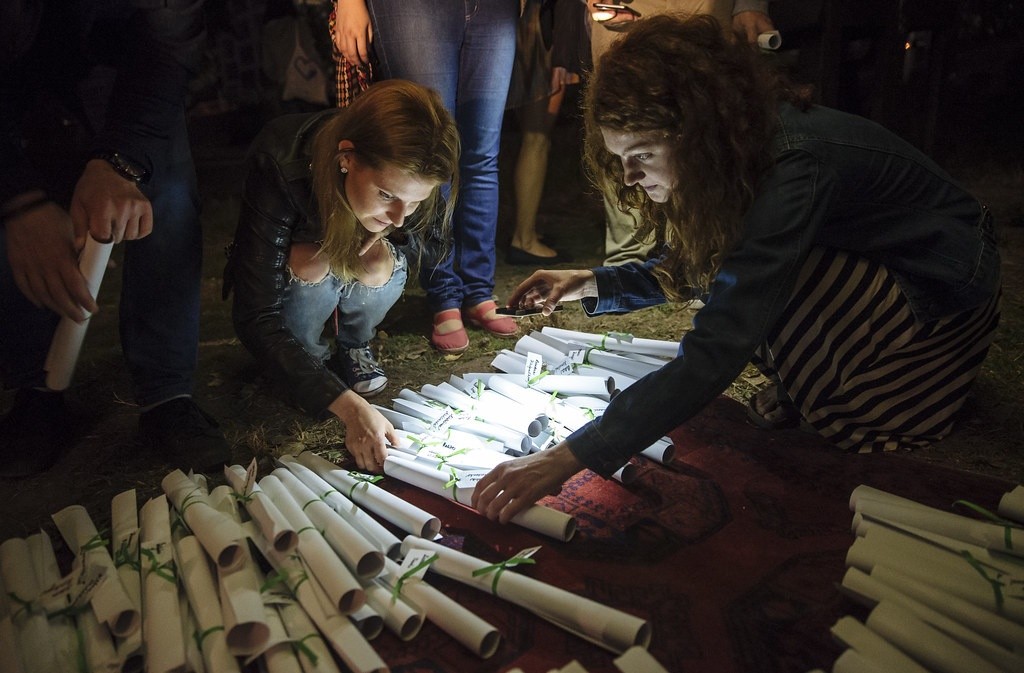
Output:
[1,380,100,479]
[338,343,389,399]
[139,395,233,475]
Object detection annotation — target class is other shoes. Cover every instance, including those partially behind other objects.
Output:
[506,232,557,248]
[432,306,472,357]
[505,245,572,265]
[469,302,519,339]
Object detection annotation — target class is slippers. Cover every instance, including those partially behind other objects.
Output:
[747,380,800,430]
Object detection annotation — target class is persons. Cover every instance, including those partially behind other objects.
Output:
[0,0,236,474]
[324,1,584,357]
[583,0,778,266]
[222,79,464,472]
[471,0,1024,527]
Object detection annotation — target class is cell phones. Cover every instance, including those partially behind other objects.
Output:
[495,305,563,316]
[592,4,641,18]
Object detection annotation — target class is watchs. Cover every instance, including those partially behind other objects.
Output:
[93,152,146,182]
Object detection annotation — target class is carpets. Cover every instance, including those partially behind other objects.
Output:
[234,390,1024,673]
